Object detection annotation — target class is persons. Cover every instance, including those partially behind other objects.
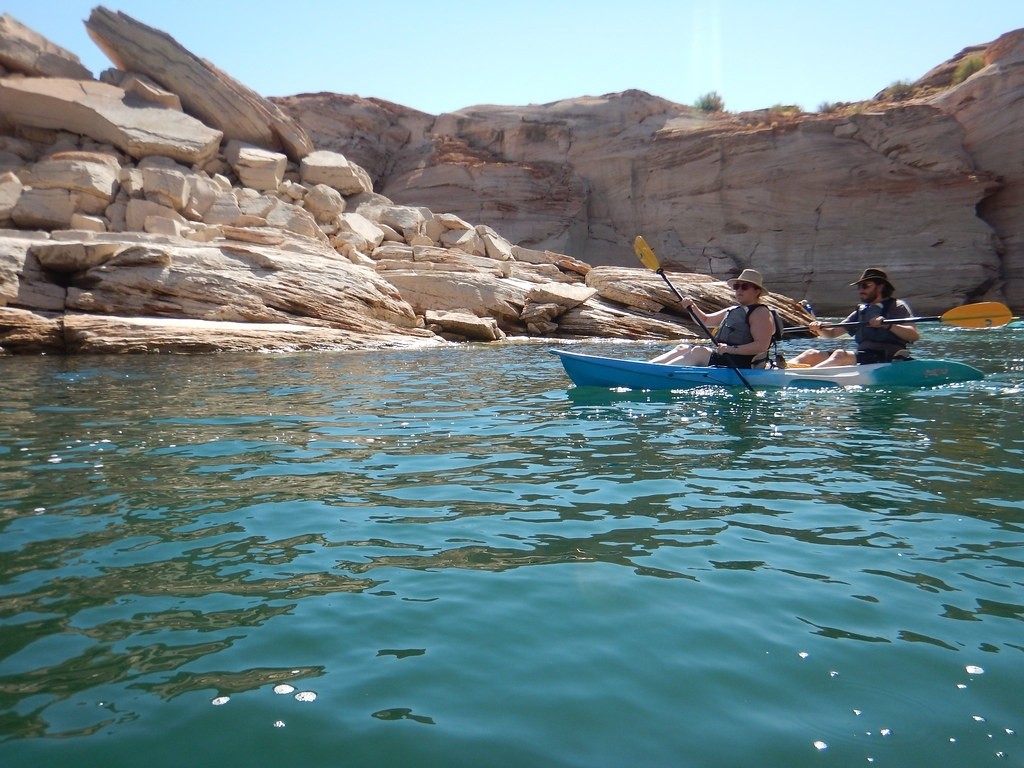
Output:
[651,268,775,370]
[788,268,920,368]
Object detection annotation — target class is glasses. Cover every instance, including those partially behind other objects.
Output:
[732,283,756,290]
[857,282,875,290]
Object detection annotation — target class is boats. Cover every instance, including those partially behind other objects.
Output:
[547,345,986,391]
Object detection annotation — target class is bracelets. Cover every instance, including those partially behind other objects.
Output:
[887,324,892,331]
[731,345,738,354]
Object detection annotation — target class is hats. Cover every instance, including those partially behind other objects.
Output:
[727,269,768,296]
[850,268,895,293]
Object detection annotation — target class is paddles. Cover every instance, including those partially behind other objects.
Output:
[632,234,758,394]
[710,299,1014,339]
